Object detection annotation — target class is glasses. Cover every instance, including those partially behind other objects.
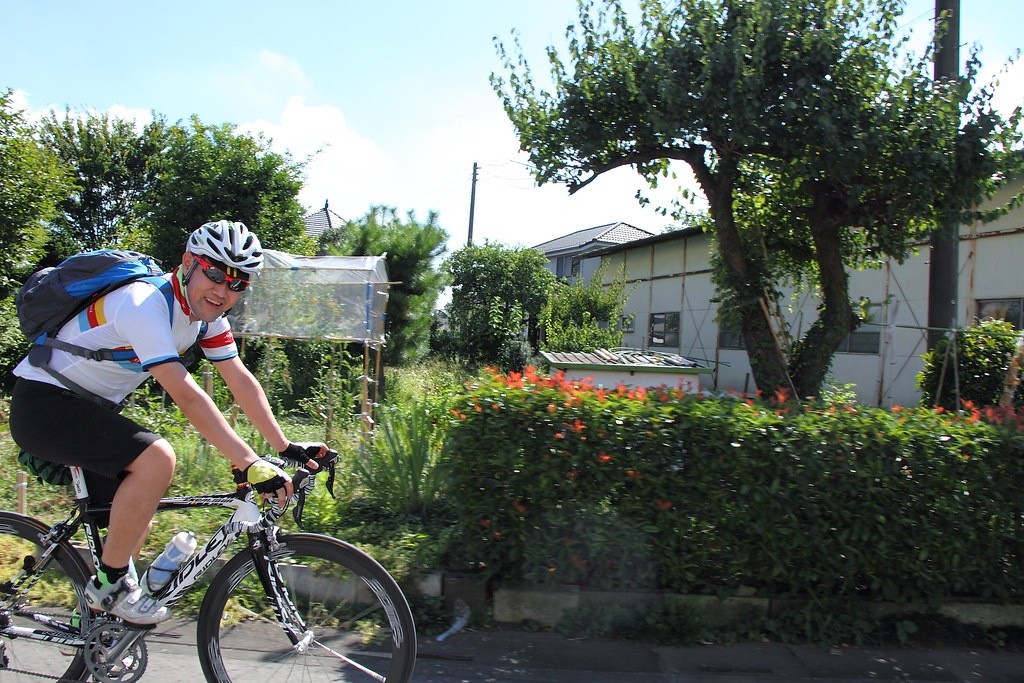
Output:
[190,252,250,292]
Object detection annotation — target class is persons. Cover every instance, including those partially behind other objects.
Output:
[10,219,327,658]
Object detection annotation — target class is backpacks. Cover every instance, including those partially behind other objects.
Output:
[16,249,209,362]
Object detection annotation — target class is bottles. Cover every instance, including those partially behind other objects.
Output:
[139,531,197,596]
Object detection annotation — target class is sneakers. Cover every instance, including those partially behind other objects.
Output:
[69,609,124,629]
[84,575,172,624]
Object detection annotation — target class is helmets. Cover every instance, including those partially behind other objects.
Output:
[186,220,264,276]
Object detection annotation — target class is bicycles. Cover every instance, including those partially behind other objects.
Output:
[0,433,418,683]
